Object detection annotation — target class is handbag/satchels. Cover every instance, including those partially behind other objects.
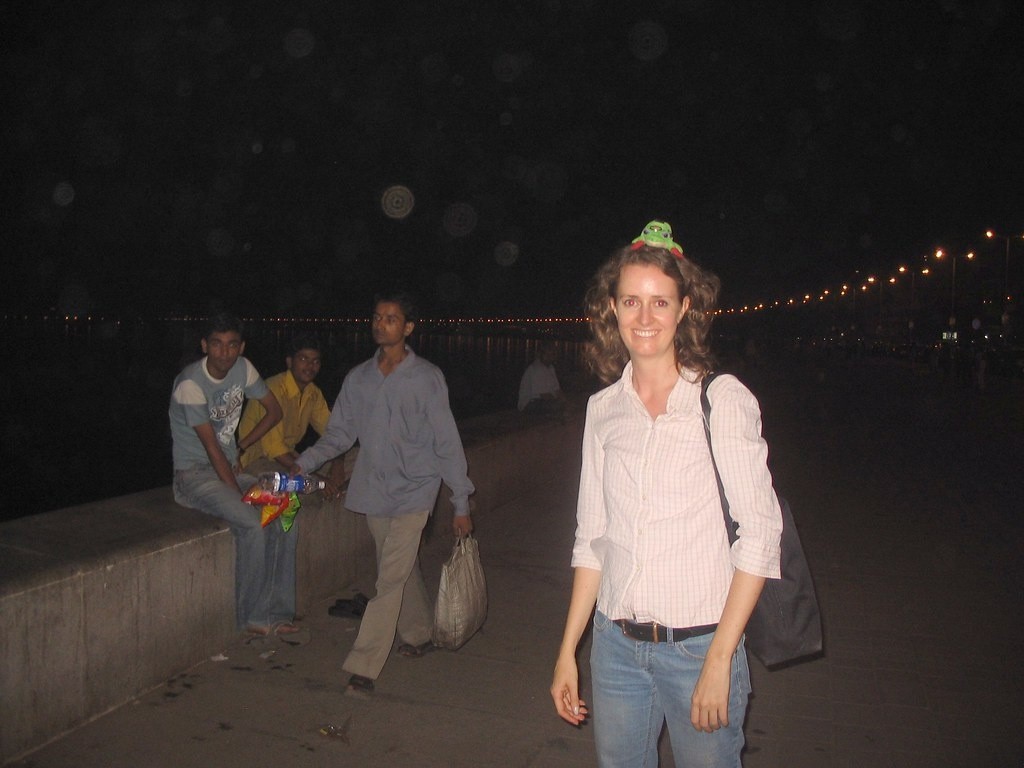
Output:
[432,527,488,650]
[700,369,822,665]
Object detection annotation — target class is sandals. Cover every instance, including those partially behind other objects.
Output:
[395,639,433,660]
[343,675,374,701]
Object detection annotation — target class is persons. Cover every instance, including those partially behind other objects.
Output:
[168,321,311,645]
[287,295,473,692]
[238,341,343,498]
[550,220,783,768]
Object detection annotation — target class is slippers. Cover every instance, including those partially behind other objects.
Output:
[249,620,311,644]
[335,593,371,608]
[228,639,294,673]
[328,601,366,620]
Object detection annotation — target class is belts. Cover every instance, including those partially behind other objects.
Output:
[613,619,719,645]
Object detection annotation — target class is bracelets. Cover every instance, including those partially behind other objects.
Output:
[236,445,245,454]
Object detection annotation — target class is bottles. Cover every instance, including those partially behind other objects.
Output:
[258,474,326,495]
